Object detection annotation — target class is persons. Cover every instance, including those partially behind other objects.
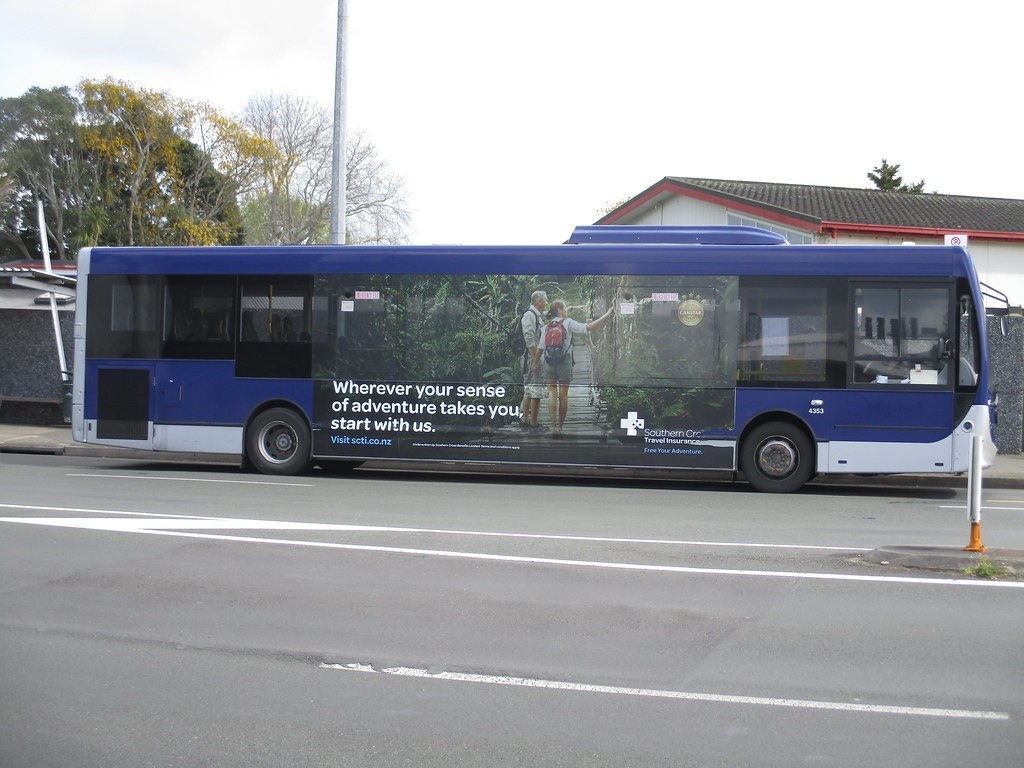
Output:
[519,291,549,433]
[531,300,614,435]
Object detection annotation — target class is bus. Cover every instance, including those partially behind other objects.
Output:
[72,227,1010,493]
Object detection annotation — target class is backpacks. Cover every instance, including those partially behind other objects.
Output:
[543,318,573,365]
[508,310,541,356]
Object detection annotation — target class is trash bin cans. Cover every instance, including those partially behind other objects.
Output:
[60,372,73,422]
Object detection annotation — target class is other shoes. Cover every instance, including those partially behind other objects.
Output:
[551,429,565,439]
[531,423,550,434]
[520,421,530,432]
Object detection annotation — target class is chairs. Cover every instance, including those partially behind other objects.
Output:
[171,304,308,350]
